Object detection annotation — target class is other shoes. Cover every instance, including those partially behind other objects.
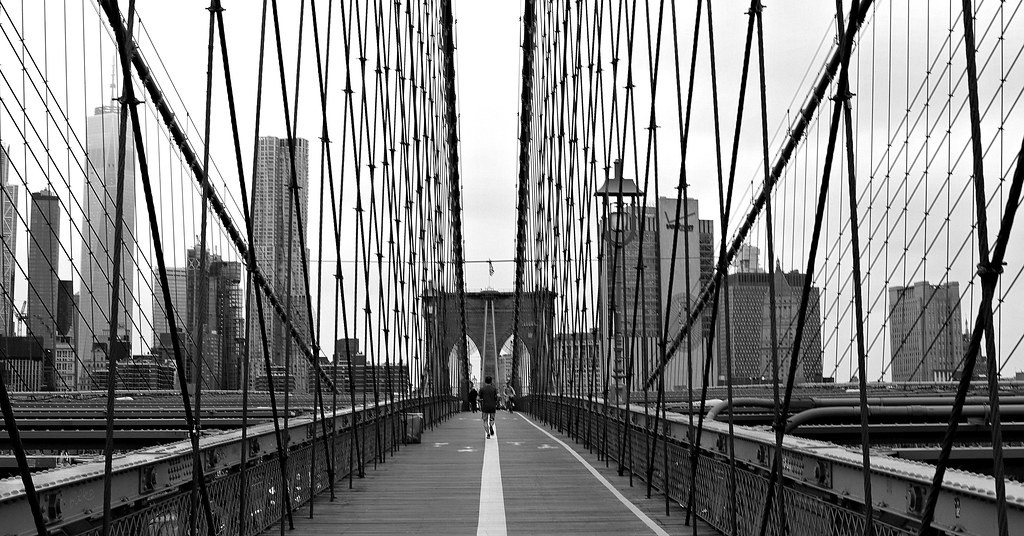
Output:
[490,426,494,435]
[487,434,490,439]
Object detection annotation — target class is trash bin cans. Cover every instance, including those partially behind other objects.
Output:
[400,413,423,444]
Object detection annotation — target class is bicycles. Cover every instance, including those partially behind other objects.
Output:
[506,395,521,414]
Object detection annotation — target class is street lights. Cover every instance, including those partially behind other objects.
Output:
[592,158,647,404]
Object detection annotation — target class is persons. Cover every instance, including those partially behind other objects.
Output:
[478,376,497,439]
[469,387,478,413]
[504,382,516,407]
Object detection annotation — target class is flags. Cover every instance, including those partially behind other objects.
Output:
[489,261,494,276]
